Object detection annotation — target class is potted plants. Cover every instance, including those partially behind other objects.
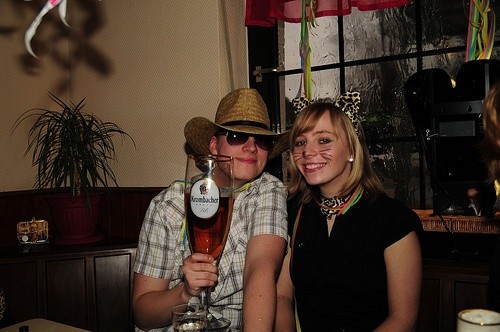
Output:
[10,96,138,246]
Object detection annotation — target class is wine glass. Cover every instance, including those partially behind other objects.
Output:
[184,153,235,329]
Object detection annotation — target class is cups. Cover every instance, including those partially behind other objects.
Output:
[457,309,500,332]
[171,302,209,332]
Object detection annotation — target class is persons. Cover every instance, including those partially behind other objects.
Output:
[131,88,288,332]
[274,102,425,332]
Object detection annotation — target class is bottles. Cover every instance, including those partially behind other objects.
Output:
[19,326,29,332]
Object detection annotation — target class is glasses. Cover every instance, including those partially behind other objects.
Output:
[215,131,277,151]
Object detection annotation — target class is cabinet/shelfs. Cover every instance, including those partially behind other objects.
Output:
[415,254,493,332]
[0,248,141,332]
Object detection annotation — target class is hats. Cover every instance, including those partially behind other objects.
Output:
[184,88,293,163]
